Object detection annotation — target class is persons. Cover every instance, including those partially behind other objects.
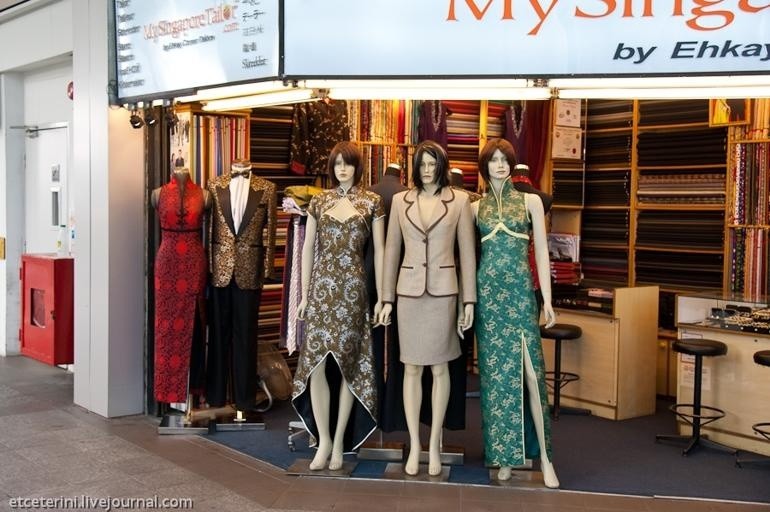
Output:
[292,138,559,489]
[150,167,211,416]
[206,159,277,421]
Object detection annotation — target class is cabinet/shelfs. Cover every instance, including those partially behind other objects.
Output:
[170,98,770,457]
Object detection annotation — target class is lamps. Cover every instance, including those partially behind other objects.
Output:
[129,106,178,130]
